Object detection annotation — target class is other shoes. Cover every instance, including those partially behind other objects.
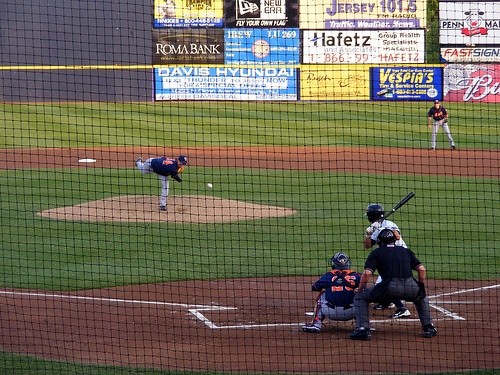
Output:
[372,303,395,310]
[350,327,371,341]
[160,206,167,211]
[301,325,320,333]
[135,157,143,167]
[424,327,437,338]
[388,308,411,319]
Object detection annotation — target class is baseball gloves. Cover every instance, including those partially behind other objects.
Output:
[178,167,184,173]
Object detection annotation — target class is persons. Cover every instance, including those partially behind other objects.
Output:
[135,155,187,211]
[426,99,456,151]
[300,253,377,332]
[363,204,407,309]
[349,229,438,341]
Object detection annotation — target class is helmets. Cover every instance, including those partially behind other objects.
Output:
[331,253,350,270]
[367,204,385,213]
[377,229,396,244]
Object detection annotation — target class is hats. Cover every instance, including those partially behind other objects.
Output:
[434,100,439,104]
[178,155,188,165]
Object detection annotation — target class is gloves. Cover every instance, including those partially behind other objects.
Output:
[366,222,381,238]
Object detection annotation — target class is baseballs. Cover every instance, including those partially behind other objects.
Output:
[207,183,212,189]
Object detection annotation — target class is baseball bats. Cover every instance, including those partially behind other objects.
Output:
[365,191,416,235]
